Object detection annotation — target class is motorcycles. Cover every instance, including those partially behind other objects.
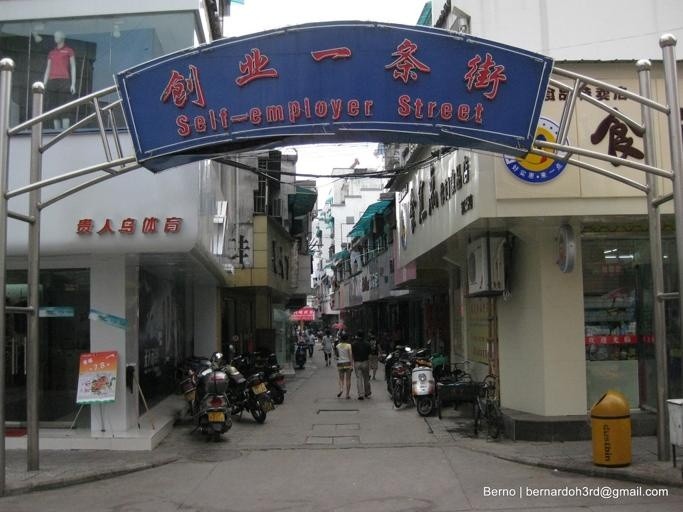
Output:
[305,337,316,357]
[376,340,436,420]
[178,333,286,443]
[293,341,309,370]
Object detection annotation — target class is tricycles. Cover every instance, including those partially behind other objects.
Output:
[434,357,484,418]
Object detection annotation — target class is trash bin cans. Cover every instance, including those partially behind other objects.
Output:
[590,391,633,467]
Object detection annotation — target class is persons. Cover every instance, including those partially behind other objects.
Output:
[352,329,371,400]
[43,31,76,129]
[299,326,400,380]
[335,334,354,399]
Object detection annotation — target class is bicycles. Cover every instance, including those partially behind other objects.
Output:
[469,371,501,439]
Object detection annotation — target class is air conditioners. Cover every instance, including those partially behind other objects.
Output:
[464,233,506,297]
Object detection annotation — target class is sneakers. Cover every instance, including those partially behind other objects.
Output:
[336,389,373,401]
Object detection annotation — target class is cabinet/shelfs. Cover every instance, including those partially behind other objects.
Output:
[584,290,638,361]
